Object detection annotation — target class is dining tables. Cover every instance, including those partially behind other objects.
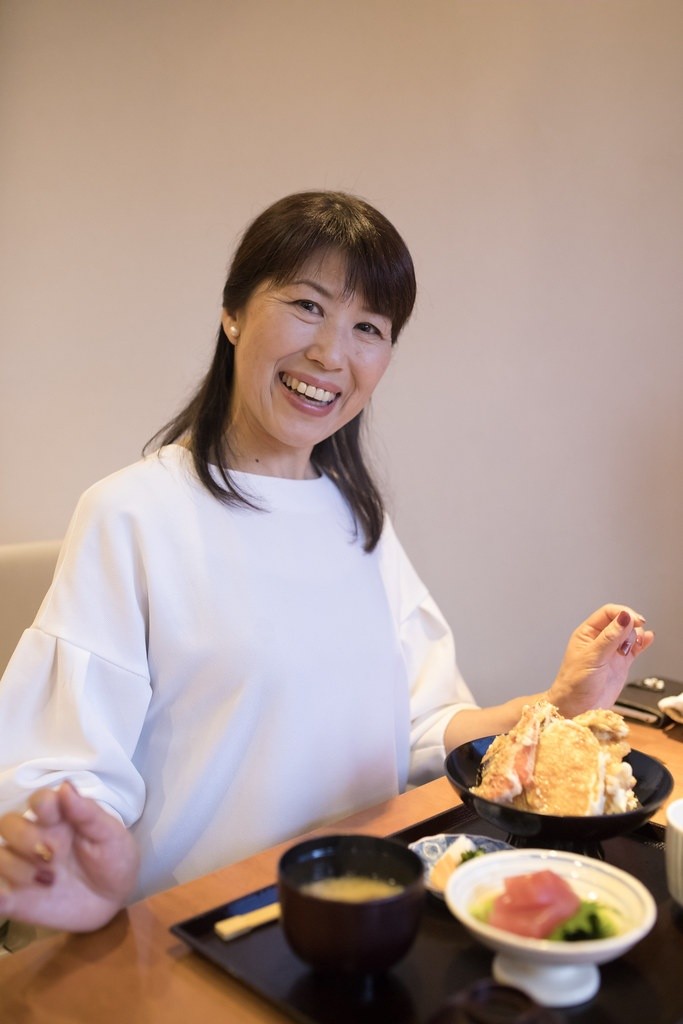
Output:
[0,718,683,1024]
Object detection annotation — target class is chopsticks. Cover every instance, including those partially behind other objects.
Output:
[214,901,281,940]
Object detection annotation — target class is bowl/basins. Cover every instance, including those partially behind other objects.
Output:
[408,834,516,902]
[278,835,426,979]
[444,849,657,1006]
[442,734,674,862]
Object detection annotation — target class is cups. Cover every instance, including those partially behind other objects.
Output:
[665,799,683,906]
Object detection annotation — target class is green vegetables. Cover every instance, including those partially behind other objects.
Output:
[544,901,616,942]
[457,849,488,862]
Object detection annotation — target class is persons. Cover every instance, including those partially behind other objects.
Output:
[1,190,656,936]
